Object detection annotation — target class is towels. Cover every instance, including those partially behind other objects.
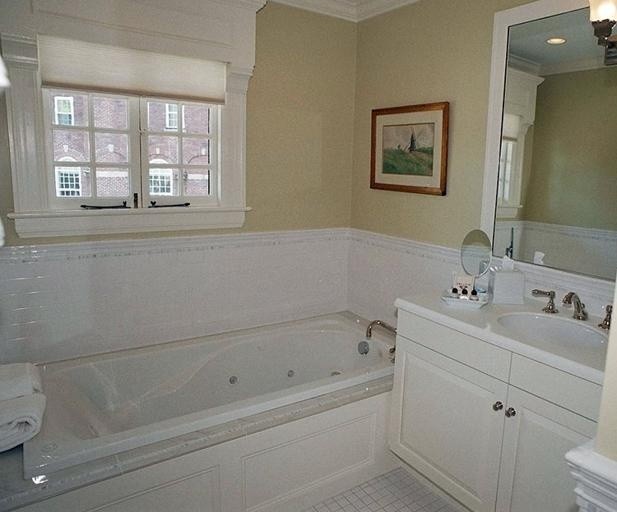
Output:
[0,359,47,453]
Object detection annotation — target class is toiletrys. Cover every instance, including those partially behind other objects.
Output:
[451,287,479,301]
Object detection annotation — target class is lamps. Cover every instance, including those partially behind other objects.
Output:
[584,1,616,54]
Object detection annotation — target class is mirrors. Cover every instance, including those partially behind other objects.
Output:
[476,0,617,286]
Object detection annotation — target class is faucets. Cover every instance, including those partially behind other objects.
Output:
[366,319,397,338]
[561,290,589,321]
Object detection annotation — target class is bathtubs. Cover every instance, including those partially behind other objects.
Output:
[22,314,397,479]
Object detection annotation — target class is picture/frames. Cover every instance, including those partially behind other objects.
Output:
[367,102,449,197]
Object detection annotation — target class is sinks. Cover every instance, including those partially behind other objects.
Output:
[496,312,608,352]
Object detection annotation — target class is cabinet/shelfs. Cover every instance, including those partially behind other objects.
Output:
[390,336,596,511]
